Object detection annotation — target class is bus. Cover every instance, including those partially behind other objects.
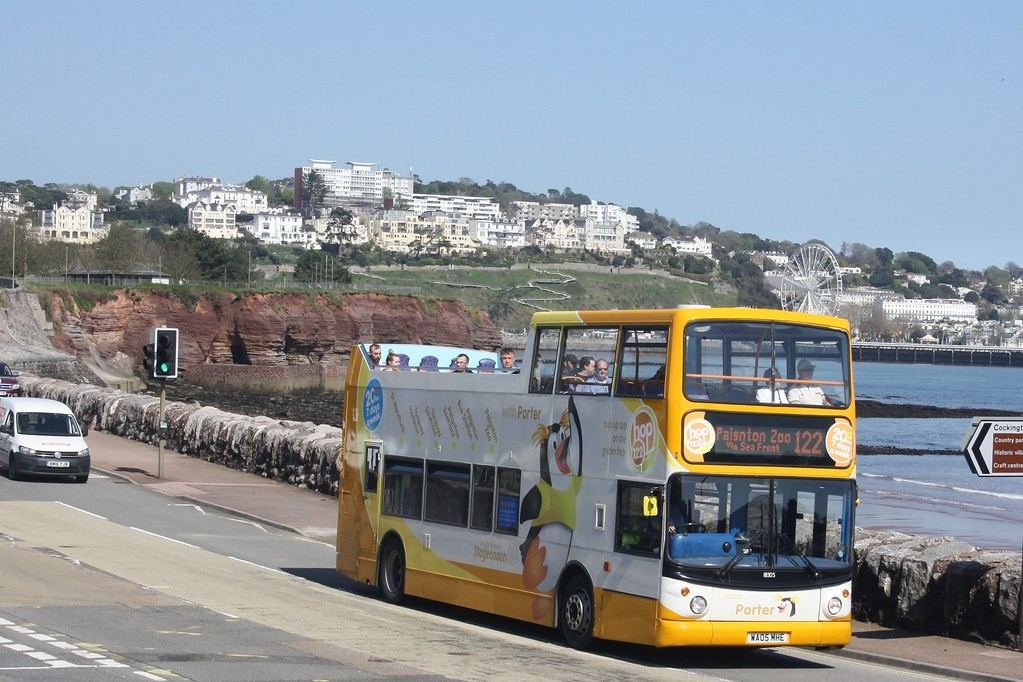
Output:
[336,302,857,655]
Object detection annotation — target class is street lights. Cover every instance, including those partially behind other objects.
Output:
[12,202,34,290]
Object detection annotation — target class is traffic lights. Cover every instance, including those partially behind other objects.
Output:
[142,343,154,375]
[154,328,179,380]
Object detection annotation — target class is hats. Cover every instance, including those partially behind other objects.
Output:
[797,359,816,370]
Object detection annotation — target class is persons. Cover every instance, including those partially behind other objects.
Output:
[644,365,666,396]
[368,343,519,375]
[19,414,31,431]
[650,493,688,537]
[788,359,833,405]
[560,353,611,394]
[755,368,789,405]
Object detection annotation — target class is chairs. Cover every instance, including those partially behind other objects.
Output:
[47,415,65,427]
[389,353,789,405]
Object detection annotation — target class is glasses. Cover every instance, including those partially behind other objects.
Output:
[597,367,608,371]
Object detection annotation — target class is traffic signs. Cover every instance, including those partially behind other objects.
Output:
[961,416,1023,478]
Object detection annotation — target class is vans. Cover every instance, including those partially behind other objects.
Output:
[0,396,91,483]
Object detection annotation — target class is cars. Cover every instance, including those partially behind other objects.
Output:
[0,363,22,397]
